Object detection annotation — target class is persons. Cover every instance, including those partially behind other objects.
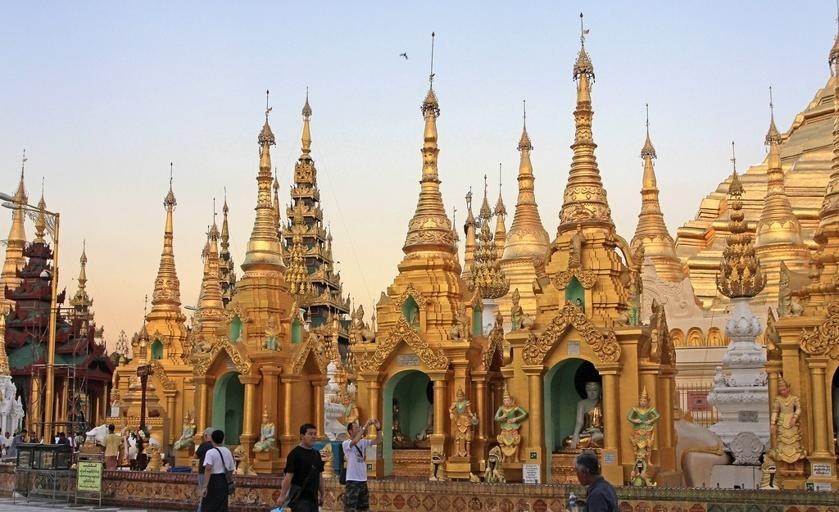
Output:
[109,313,283,477]
[342,392,360,424]
[126,426,151,471]
[411,305,421,323]
[452,300,475,342]
[200,430,235,512]
[769,371,809,464]
[509,287,523,331]
[194,426,216,491]
[30,431,40,443]
[576,298,584,313]
[55,431,84,452]
[5,429,27,458]
[625,385,661,487]
[342,417,383,512]
[494,310,506,338]
[574,450,619,512]
[560,371,604,452]
[390,387,529,484]
[102,424,123,469]
[276,424,326,512]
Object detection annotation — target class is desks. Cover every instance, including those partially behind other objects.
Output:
[14,469,71,508]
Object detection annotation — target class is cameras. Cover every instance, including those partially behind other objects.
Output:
[370,419,376,424]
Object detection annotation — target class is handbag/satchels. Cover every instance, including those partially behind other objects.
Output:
[339,469,347,484]
[225,468,235,495]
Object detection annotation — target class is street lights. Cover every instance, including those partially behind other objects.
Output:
[0,192,65,449]
[185,301,197,315]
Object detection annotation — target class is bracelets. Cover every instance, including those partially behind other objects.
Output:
[377,428,381,431]
[362,427,365,430]
